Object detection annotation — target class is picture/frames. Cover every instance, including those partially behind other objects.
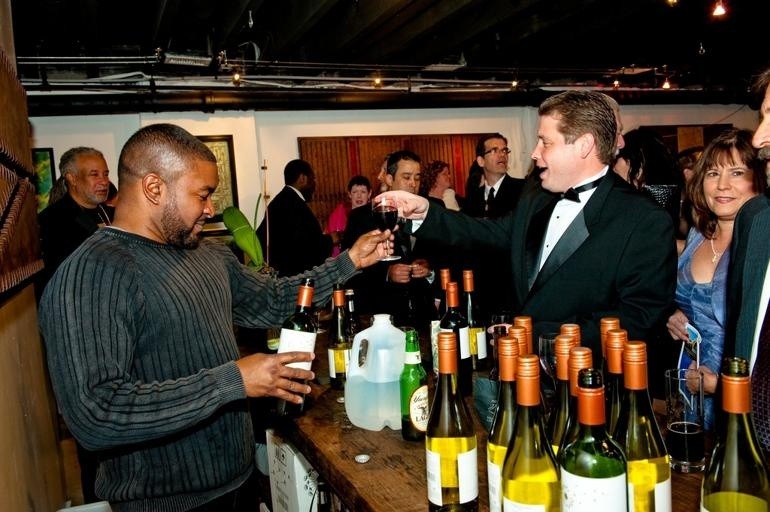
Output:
[31,147,56,214]
[200,229,245,266]
[194,134,239,223]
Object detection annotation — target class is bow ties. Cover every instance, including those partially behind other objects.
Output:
[553,187,580,202]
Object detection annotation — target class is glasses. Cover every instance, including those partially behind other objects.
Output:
[483,147,511,157]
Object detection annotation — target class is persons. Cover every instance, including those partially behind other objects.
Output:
[40,121,397,510]
[33,136,124,278]
[249,66,770,511]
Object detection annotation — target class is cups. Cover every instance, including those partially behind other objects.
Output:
[662,366,706,472]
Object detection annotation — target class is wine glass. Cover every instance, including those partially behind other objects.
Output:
[368,191,404,264]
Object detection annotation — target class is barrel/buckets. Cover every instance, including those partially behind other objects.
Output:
[344,314,406,432]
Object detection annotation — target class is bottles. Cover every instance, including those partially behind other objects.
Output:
[553,317,628,512]
[486,316,559,511]
[269,275,317,416]
[400,326,429,442]
[438,265,490,396]
[327,281,354,389]
[421,328,482,512]
[598,317,671,511]
[699,356,765,511]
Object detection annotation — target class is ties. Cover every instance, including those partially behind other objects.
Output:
[752,299,770,452]
[487,187,494,210]
